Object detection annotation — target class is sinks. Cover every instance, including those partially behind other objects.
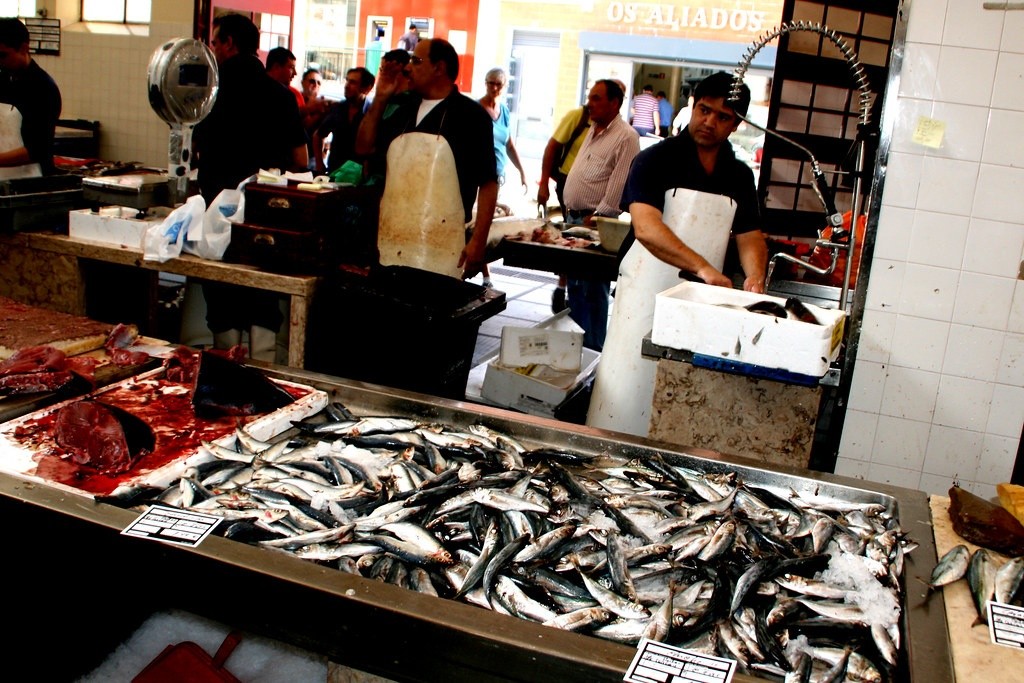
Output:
[763,281,854,318]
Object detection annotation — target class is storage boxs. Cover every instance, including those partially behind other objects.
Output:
[229,181,350,274]
[68,204,168,256]
[478,343,602,419]
[651,281,845,376]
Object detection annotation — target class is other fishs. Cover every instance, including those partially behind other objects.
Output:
[969,548,996,626]
[916,545,969,592]
[156,415,918,682]
[991,555,1024,607]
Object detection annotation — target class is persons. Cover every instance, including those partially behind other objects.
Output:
[0,18,60,178]
[193,14,693,400]
[583,72,768,440]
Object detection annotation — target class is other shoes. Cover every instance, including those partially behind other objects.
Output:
[552,288,565,314]
[482,281,494,288]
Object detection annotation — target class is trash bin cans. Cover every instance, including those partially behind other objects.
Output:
[0,175,85,233]
[348,266,507,401]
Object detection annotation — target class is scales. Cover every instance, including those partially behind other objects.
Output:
[82,36,219,221]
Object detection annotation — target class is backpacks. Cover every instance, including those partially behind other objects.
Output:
[547,104,590,176]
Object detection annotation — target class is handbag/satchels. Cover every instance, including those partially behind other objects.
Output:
[185,174,255,261]
[143,194,206,263]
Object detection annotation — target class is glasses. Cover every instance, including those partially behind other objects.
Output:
[309,79,321,85]
[411,55,428,65]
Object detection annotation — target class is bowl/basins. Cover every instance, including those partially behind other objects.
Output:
[590,217,630,252]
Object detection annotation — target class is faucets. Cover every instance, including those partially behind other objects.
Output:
[767,242,840,276]
